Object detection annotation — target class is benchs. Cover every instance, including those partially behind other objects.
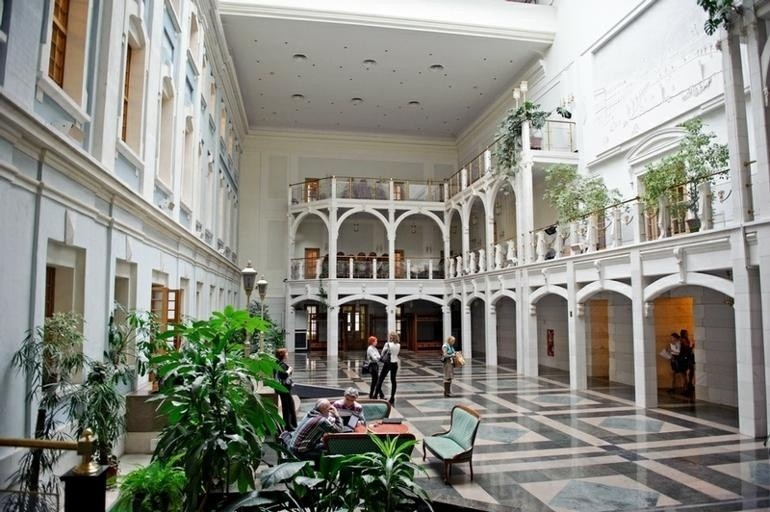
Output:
[420,404,483,486]
[315,433,418,475]
[355,400,390,418]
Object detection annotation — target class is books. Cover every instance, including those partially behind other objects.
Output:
[382,418,401,424]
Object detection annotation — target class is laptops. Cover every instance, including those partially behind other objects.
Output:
[340,412,359,433]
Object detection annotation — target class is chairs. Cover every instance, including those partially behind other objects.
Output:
[278,431,310,460]
[323,250,391,278]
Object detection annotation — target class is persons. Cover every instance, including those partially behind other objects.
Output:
[274,347,297,431]
[679,329,695,391]
[373,331,401,407]
[439,335,463,397]
[665,332,689,394]
[331,387,367,425]
[287,399,344,470]
[365,336,388,400]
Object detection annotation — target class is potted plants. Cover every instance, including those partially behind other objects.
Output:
[66,362,130,491]
[642,112,728,233]
[491,100,573,181]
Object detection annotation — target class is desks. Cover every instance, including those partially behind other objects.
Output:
[351,418,410,434]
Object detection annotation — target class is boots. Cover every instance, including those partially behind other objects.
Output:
[445,382,454,397]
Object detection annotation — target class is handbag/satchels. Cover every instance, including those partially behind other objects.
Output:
[363,360,379,374]
[380,346,392,364]
[453,351,466,368]
[660,350,672,360]
[441,353,451,363]
[273,376,294,393]
